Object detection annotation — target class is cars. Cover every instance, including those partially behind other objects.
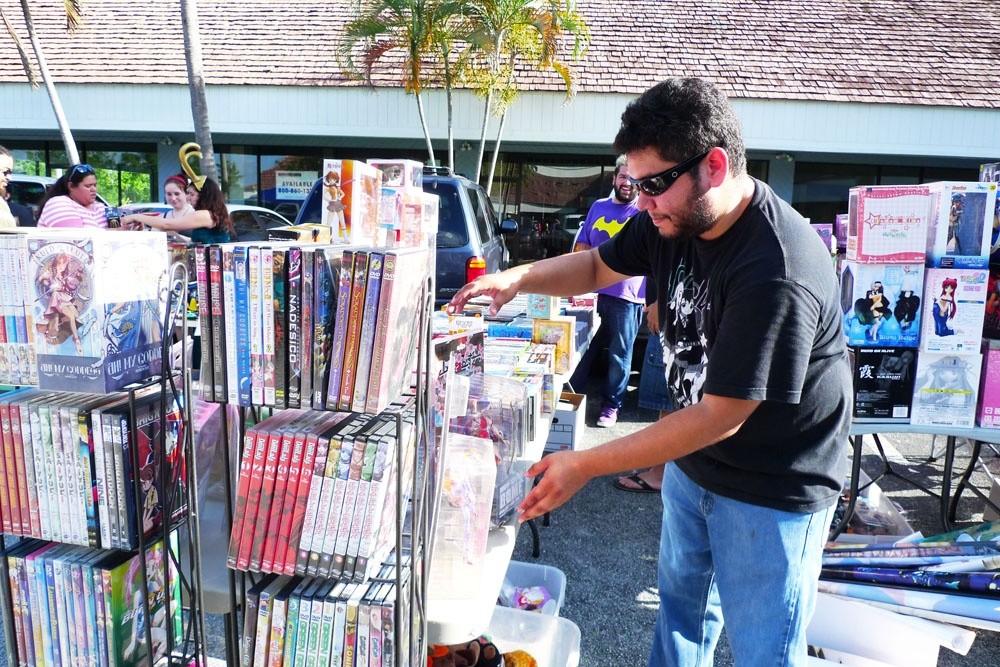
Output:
[223,202,296,249]
[295,164,519,318]
[552,214,588,249]
[3,174,174,228]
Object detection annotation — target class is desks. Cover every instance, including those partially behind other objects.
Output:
[428,405,552,649]
[822,415,1000,544]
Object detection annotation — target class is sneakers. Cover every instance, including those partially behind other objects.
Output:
[597,400,621,427]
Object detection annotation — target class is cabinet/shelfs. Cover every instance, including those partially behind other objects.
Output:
[1,258,214,666]
[216,265,431,667]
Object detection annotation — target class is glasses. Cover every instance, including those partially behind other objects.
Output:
[627,142,724,198]
[68,163,95,182]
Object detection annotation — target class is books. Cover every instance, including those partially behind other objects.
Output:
[0,229,604,667]
[803,157,1000,437]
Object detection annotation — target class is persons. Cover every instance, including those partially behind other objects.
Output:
[151,176,197,235]
[574,150,651,427]
[121,177,233,244]
[34,164,145,233]
[616,272,674,494]
[1,145,21,228]
[450,76,858,667]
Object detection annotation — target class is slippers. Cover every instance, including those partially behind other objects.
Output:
[613,472,662,494]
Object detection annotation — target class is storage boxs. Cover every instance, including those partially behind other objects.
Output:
[802,147,998,428]
[541,391,588,454]
[485,603,584,667]
[264,146,440,252]
[491,559,568,617]
